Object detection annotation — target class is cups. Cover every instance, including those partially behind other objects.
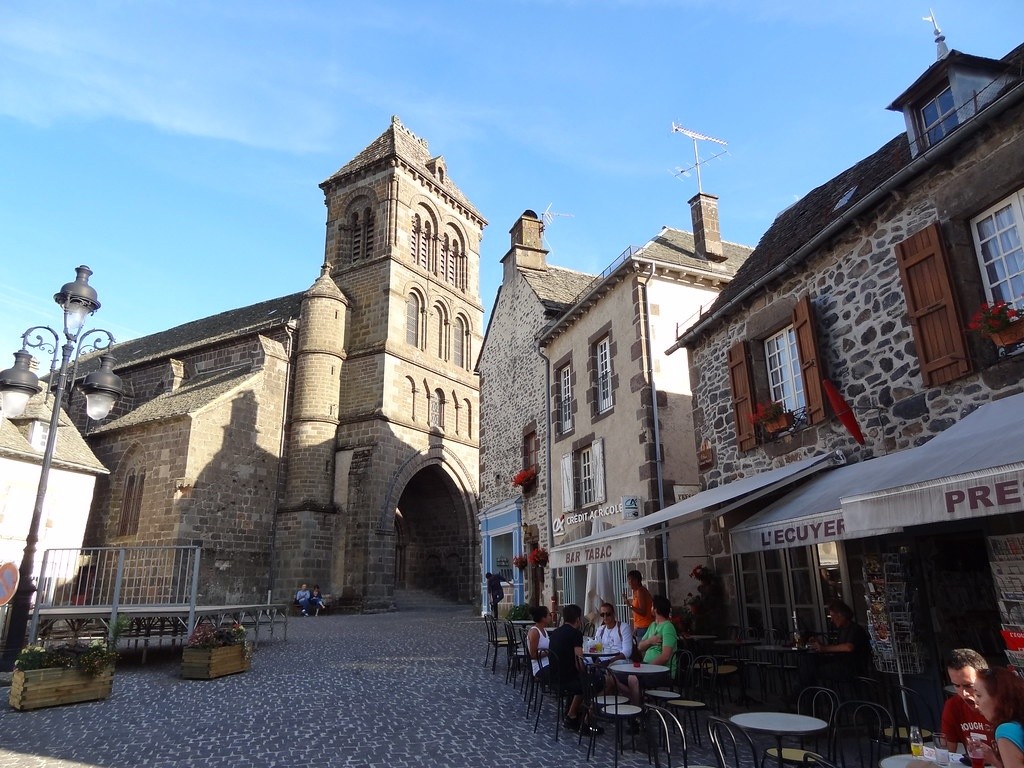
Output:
[967,736,985,768]
[932,733,949,764]
[583,636,614,653]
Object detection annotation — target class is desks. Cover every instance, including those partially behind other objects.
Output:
[880,752,972,768]
[545,627,558,631]
[729,712,829,768]
[583,648,620,663]
[754,644,809,666]
[609,663,670,757]
[678,633,718,641]
[508,620,536,628]
[714,639,762,681]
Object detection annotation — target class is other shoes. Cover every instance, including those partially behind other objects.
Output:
[490,602,494,611]
[625,718,640,736]
[493,618,498,621]
[561,717,604,736]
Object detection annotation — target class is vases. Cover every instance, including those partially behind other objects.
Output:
[763,412,794,434]
[990,319,1024,347]
[8,667,116,710]
[180,643,251,681]
[522,479,535,493]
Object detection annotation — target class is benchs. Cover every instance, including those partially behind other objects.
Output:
[327,593,364,615]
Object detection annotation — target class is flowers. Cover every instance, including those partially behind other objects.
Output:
[746,402,783,424]
[512,468,535,487]
[529,547,549,568]
[187,622,248,649]
[511,555,528,570]
[14,637,121,681]
[669,563,713,637]
[967,300,1024,334]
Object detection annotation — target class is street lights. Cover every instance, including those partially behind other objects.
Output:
[0,265,126,673]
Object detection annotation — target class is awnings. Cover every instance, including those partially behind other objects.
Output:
[547,393,1024,570]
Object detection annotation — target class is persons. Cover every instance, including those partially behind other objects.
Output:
[809,602,880,727]
[528,606,550,678]
[939,648,1024,768]
[624,570,656,647]
[628,595,677,734]
[297,584,325,617]
[549,602,633,735]
[486,573,514,620]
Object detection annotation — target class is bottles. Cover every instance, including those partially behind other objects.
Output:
[910,726,923,757]
[632,645,640,667]
[794,629,801,647]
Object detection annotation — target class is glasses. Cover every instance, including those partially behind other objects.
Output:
[986,669,996,679]
[544,613,552,616]
[600,612,611,617]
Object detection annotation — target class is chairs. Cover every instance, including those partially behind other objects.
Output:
[713,624,840,702]
[314,605,331,616]
[643,704,837,768]
[757,677,932,768]
[292,589,312,616]
[484,614,660,768]
